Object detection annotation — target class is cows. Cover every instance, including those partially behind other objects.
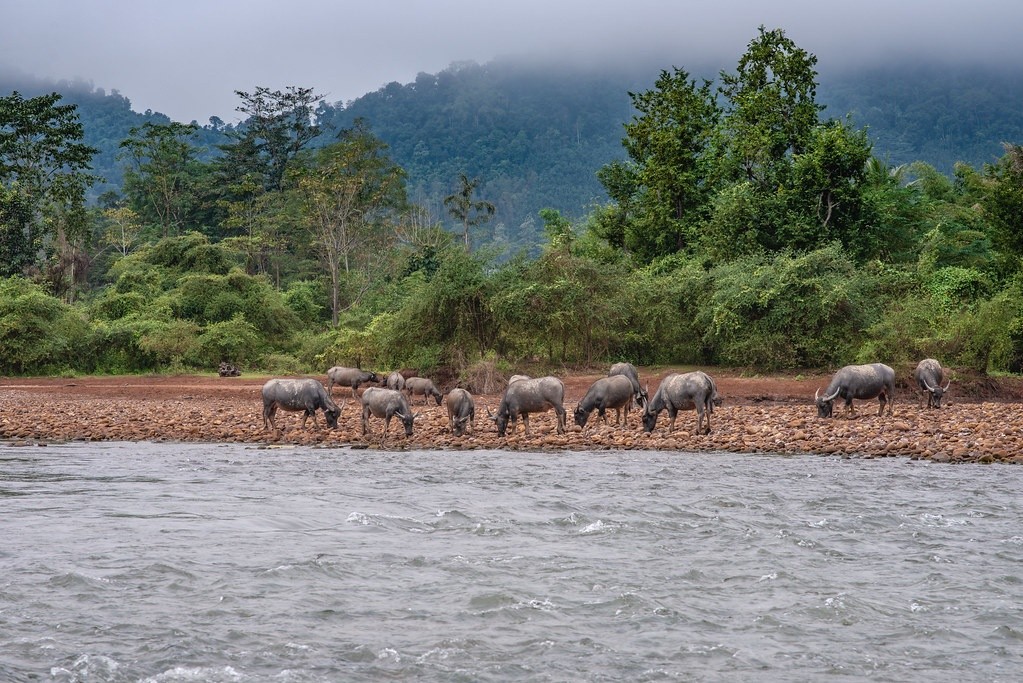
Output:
[402,373,442,407]
[641,370,716,435]
[484,373,568,437]
[261,378,340,429]
[383,369,405,392]
[913,357,950,412]
[815,363,896,419]
[574,374,634,435]
[446,388,475,437]
[362,386,420,438]
[323,364,378,397]
[607,363,648,410]
